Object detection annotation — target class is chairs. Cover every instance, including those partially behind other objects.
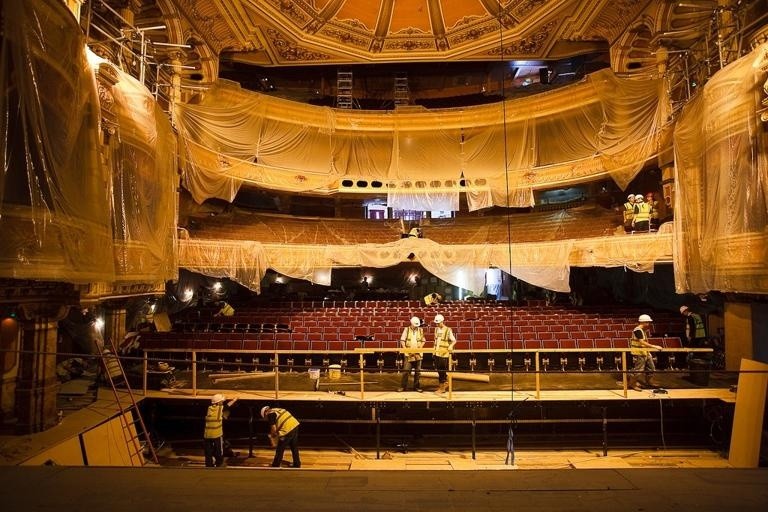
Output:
[130,298,689,379]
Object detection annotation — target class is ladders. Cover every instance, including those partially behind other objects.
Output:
[95,337,158,466]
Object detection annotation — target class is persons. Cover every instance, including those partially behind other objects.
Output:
[431,315,457,394]
[628,314,663,392]
[622,191,666,233]
[362,276,368,287]
[397,316,426,392]
[260,406,301,468]
[408,228,421,239]
[422,292,442,308]
[213,301,235,318]
[99,349,122,389]
[679,305,706,348]
[56,357,88,384]
[413,276,420,288]
[202,393,239,467]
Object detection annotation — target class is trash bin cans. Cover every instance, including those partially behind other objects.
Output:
[687,357,712,386]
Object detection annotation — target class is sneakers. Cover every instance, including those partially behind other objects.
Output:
[630,384,642,391]
[413,387,424,392]
[646,382,659,387]
[398,386,408,392]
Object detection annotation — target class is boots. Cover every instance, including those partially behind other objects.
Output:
[434,380,449,395]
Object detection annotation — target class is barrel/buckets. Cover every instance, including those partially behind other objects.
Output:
[329,364,341,379]
[308,369,320,380]
[688,357,715,384]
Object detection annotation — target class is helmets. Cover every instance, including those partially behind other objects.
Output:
[680,306,689,314]
[260,405,270,419]
[102,349,111,356]
[211,394,225,404]
[410,317,421,327]
[638,314,653,322]
[627,192,654,199]
[433,314,445,323]
